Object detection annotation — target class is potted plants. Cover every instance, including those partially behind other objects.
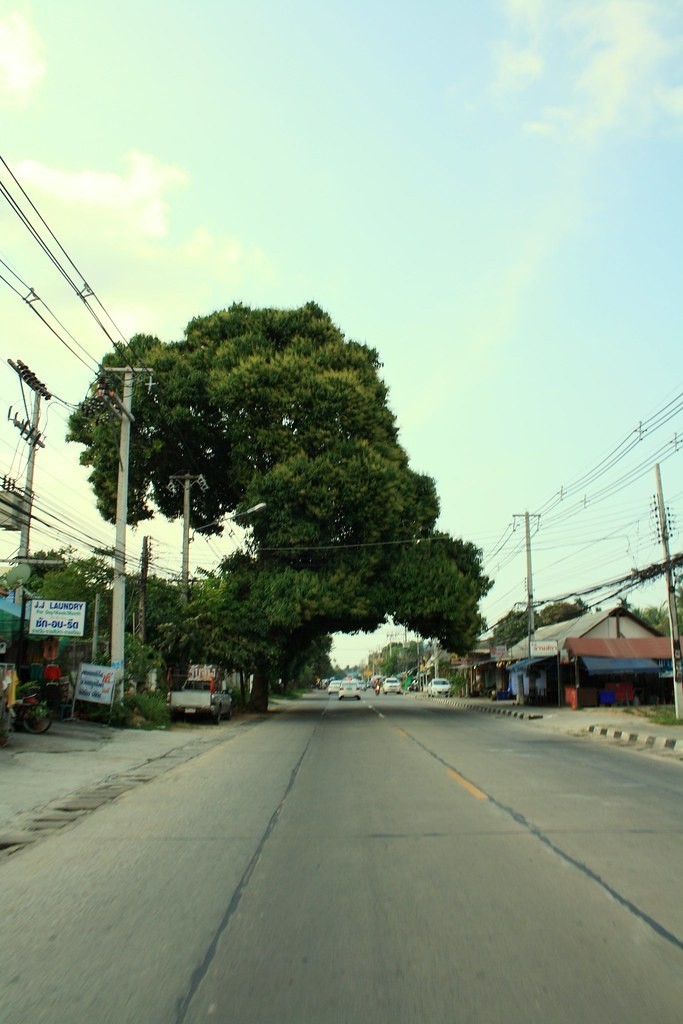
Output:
[20,680,41,703]
[29,700,48,730]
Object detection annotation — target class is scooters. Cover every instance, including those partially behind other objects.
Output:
[8,687,52,735]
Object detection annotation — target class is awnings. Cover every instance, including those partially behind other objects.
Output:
[507,657,545,672]
[0,599,48,641]
[582,656,659,675]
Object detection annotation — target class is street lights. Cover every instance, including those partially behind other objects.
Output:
[180,503,268,608]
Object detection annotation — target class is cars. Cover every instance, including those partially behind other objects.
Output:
[357,682,366,692]
[338,681,361,700]
[426,678,452,698]
[382,677,401,694]
[326,680,342,695]
[367,682,375,689]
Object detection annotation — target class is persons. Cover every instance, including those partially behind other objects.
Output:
[412,680,418,690]
[375,679,381,686]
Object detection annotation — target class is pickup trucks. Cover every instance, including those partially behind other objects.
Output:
[165,677,234,721]
